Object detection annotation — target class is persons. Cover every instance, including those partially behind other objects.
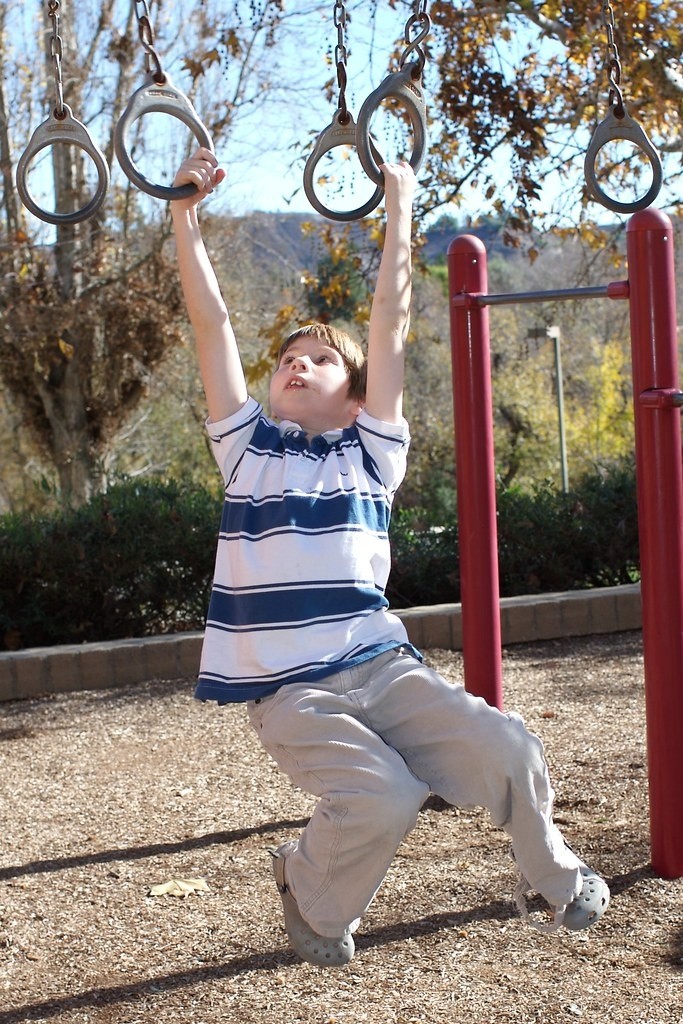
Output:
[169,145,610,967]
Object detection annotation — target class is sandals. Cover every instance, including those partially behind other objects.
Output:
[267,840,354,966]
[549,864,610,930]
